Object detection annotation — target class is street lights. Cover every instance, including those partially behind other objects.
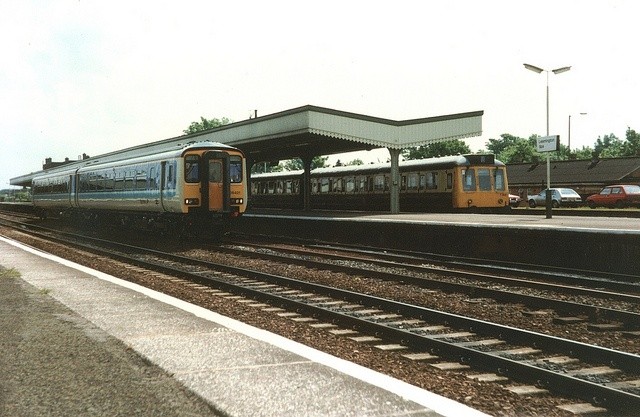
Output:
[568,112,587,150]
[523,61,572,219]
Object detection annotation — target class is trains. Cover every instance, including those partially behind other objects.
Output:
[246,155,510,208]
[31,140,247,239]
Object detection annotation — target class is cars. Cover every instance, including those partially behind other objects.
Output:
[528,186,582,208]
[508,194,521,206]
[585,184,639,208]
